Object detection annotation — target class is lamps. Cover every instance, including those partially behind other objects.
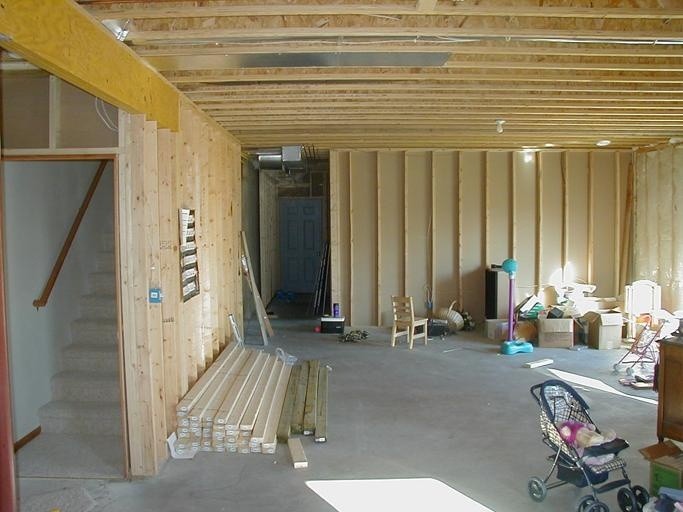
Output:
[492,119,504,135]
[522,151,531,163]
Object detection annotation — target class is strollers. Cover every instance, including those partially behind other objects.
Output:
[527,379,648,512]
[612,313,679,375]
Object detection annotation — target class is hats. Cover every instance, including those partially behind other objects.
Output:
[559,420,584,445]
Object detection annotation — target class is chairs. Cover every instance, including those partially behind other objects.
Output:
[389,294,428,350]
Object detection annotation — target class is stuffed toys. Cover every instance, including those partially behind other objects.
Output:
[557,420,602,446]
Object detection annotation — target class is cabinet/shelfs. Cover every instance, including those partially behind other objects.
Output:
[654,328,682,443]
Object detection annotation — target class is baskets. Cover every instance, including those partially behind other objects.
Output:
[435,301,465,333]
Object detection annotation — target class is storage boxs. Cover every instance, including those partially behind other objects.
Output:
[580,309,622,351]
[637,439,682,499]
[319,315,344,334]
[536,316,573,349]
